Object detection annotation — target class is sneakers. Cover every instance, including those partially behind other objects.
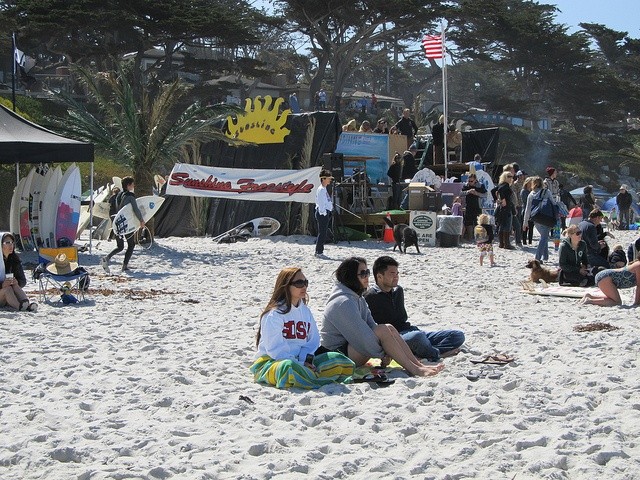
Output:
[27,302,38,312]
[19,299,29,311]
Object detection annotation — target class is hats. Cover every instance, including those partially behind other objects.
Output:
[320,176,334,178]
[546,168,556,177]
[521,170,528,175]
[515,170,523,176]
[619,184,629,192]
[45,252,78,275]
[409,144,417,152]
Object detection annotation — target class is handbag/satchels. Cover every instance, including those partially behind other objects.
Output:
[529,188,560,228]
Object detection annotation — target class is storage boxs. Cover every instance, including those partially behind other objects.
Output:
[402,182,435,210]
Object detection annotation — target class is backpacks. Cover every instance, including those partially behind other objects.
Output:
[474,225,489,243]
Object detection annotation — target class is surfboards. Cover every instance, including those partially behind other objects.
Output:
[74,183,115,240]
[327,225,371,244]
[112,176,134,194]
[112,196,166,236]
[212,217,281,243]
[9,162,82,253]
[153,174,167,194]
[517,277,621,298]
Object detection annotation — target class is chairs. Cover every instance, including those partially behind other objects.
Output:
[37,245,90,306]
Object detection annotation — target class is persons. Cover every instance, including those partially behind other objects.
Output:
[387,153,402,210]
[102,176,145,273]
[577,208,615,266]
[543,167,561,237]
[520,178,534,248]
[248,268,356,391]
[319,256,445,377]
[0,232,38,312]
[400,143,417,211]
[460,174,488,238]
[473,214,497,266]
[512,170,527,224]
[465,153,484,172]
[390,108,418,147]
[431,114,445,144]
[578,186,597,218]
[358,120,373,133]
[446,125,462,162]
[342,119,358,132]
[362,256,465,362]
[373,118,389,134]
[609,245,627,268]
[504,162,518,183]
[490,171,519,249]
[315,169,334,260]
[522,176,561,261]
[559,184,578,235]
[616,184,633,229]
[558,223,595,288]
[319,88,327,108]
[580,250,640,308]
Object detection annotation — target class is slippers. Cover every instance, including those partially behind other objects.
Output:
[470,356,508,364]
[371,373,395,386]
[488,369,503,378]
[482,354,514,362]
[354,372,388,383]
[465,368,483,380]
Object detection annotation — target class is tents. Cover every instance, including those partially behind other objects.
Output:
[601,196,631,229]
[565,206,584,227]
[569,184,612,211]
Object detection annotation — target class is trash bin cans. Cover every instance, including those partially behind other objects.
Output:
[435,215,464,247]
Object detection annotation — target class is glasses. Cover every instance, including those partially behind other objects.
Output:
[468,179,475,183]
[2,241,14,245]
[507,176,513,178]
[572,231,582,236]
[289,279,308,288]
[356,269,370,278]
